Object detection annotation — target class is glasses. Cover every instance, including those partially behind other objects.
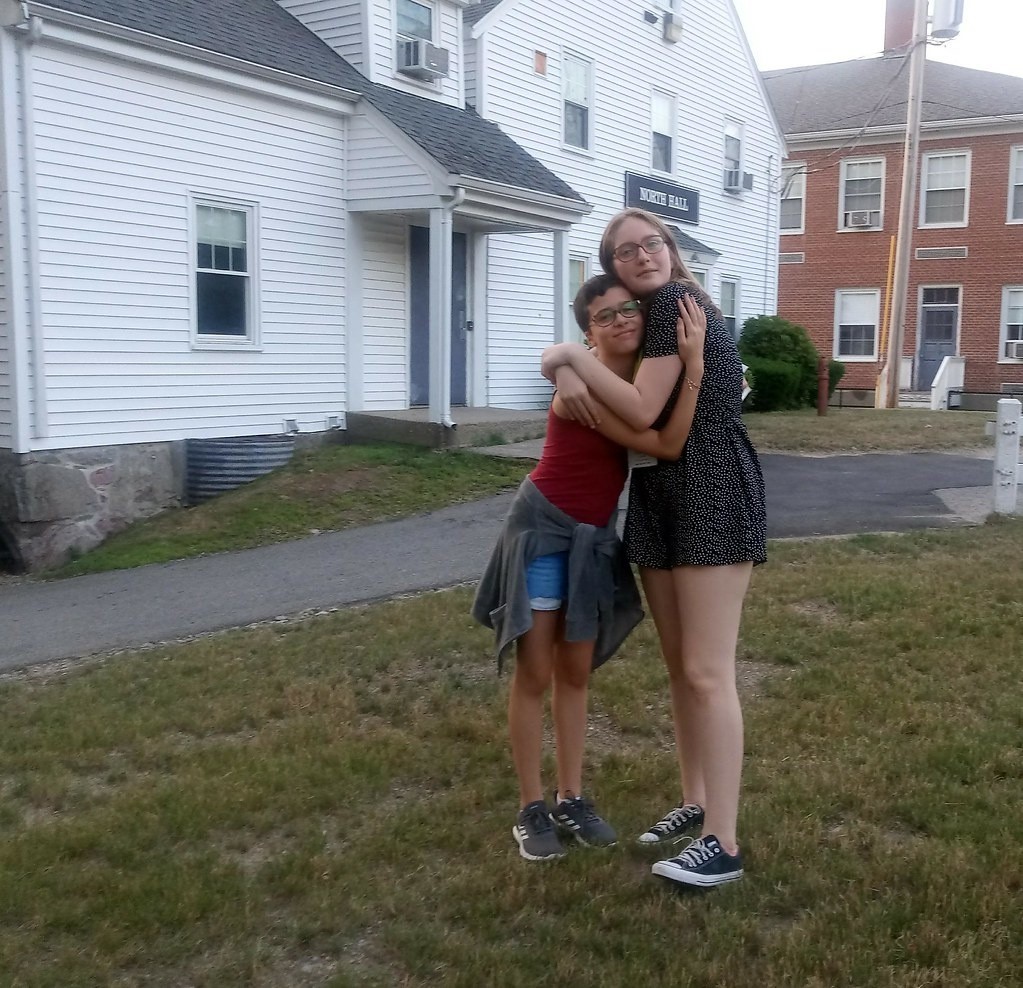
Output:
[587,299,641,327]
[612,235,667,263]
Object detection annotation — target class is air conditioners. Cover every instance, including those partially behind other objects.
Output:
[729,170,754,191]
[849,210,871,227]
[1007,342,1023,359]
[397,38,449,80]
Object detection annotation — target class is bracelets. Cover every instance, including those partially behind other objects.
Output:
[685,377,701,390]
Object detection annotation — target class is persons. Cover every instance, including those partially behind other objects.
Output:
[470,275,708,861]
[540,208,769,887]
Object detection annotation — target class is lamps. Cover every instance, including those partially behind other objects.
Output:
[664,13,682,43]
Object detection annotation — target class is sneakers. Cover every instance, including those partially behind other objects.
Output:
[635,805,705,847]
[549,790,620,850]
[650,834,745,886]
[512,800,566,860]
